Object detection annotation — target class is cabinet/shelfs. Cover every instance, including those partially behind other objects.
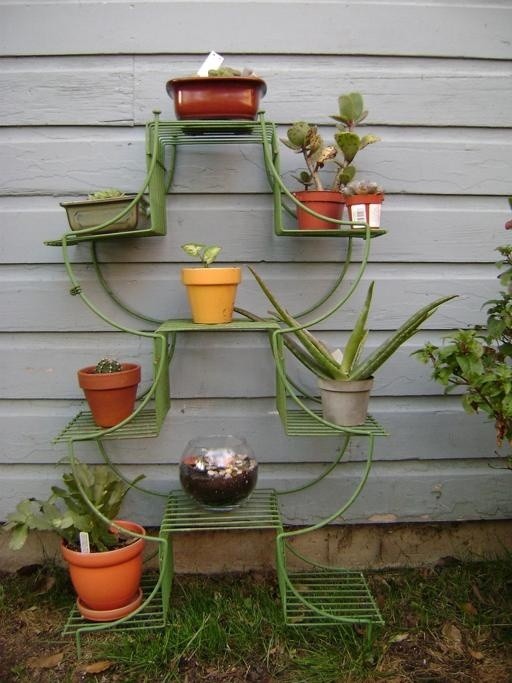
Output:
[45,119,387,659]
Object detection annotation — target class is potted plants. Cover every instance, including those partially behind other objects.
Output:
[2,457,147,621]
[60,188,150,234]
[77,358,141,428]
[279,90,382,237]
[179,434,260,512]
[234,265,459,427]
[180,242,240,324]
[339,179,385,230]
[165,66,268,135]
